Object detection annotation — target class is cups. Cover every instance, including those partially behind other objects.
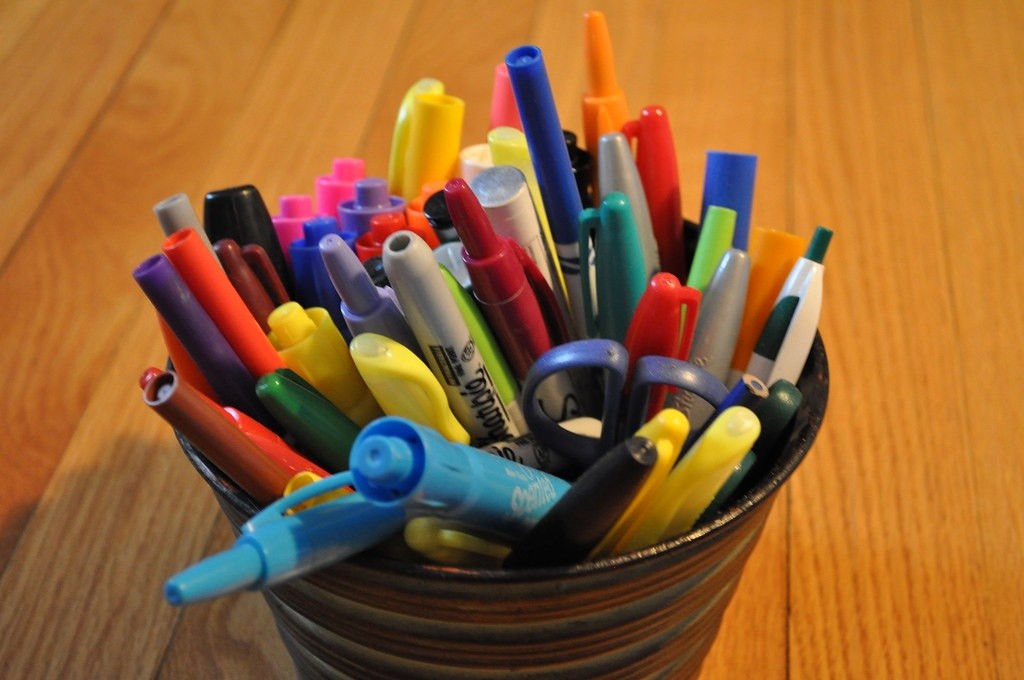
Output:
[166,219,830,680]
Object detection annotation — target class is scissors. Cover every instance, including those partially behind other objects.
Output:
[522,337,730,470]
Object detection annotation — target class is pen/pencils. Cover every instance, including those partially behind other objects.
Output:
[132,9,835,608]
